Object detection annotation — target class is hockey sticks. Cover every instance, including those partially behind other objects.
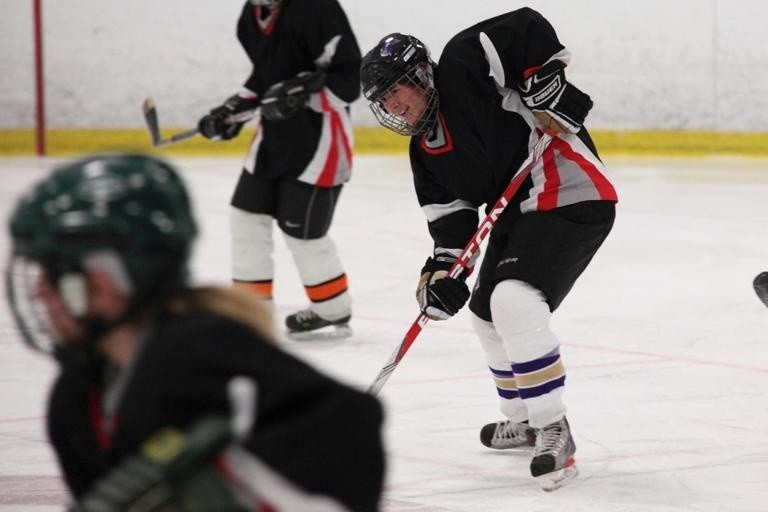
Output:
[142,95,297,148]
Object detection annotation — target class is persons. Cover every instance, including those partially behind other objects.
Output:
[360,7,618,477]
[2,156,386,512]
[199,0,364,331]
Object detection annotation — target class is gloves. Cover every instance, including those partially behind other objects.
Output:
[514,59,593,141]
[260,71,312,120]
[416,253,469,321]
[198,92,257,139]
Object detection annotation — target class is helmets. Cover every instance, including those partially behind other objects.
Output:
[6,153,197,355]
[358,31,440,138]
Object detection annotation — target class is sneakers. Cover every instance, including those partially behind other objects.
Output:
[286,309,351,332]
[530,416,577,476]
[480,420,535,448]
